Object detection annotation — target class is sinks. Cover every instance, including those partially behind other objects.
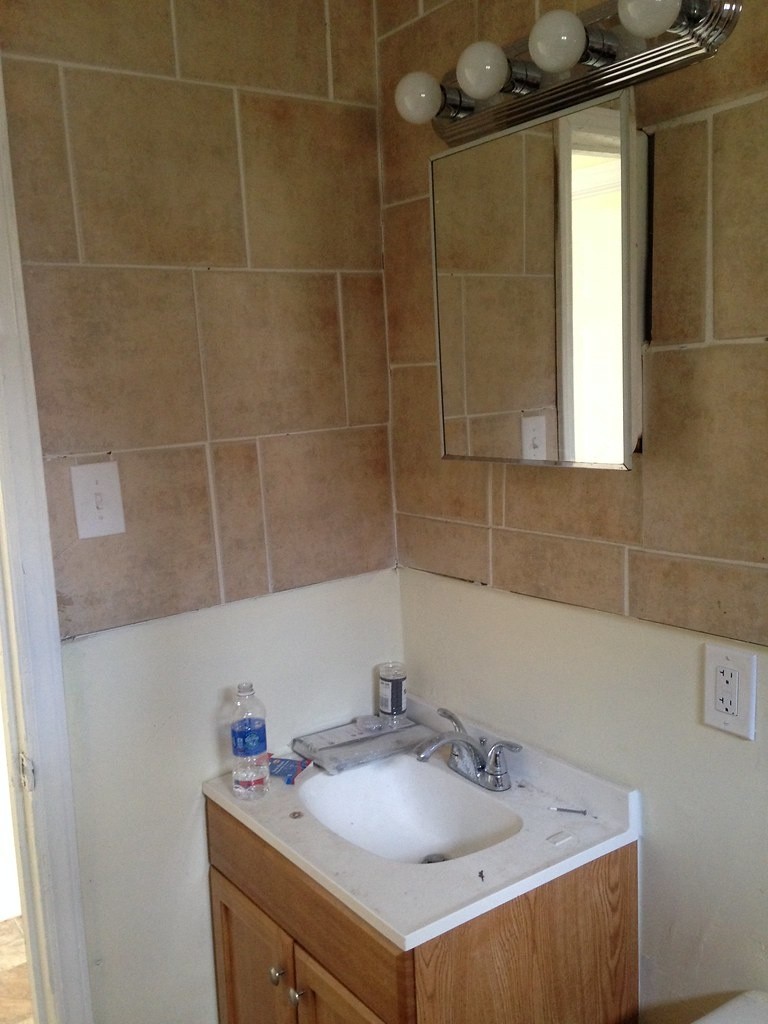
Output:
[300,753,523,864]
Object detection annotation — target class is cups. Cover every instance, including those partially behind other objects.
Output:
[377,662,407,720]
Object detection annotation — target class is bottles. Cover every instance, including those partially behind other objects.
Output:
[231,681,270,801]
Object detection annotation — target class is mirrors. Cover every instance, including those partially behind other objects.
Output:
[430,86,650,470]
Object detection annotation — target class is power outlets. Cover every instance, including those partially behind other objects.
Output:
[701,641,758,739]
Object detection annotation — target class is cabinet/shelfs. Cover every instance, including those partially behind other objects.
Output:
[204,694,646,1024]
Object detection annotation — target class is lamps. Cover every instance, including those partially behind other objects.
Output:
[394,0,744,148]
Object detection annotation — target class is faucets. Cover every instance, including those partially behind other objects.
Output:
[415,730,488,788]
